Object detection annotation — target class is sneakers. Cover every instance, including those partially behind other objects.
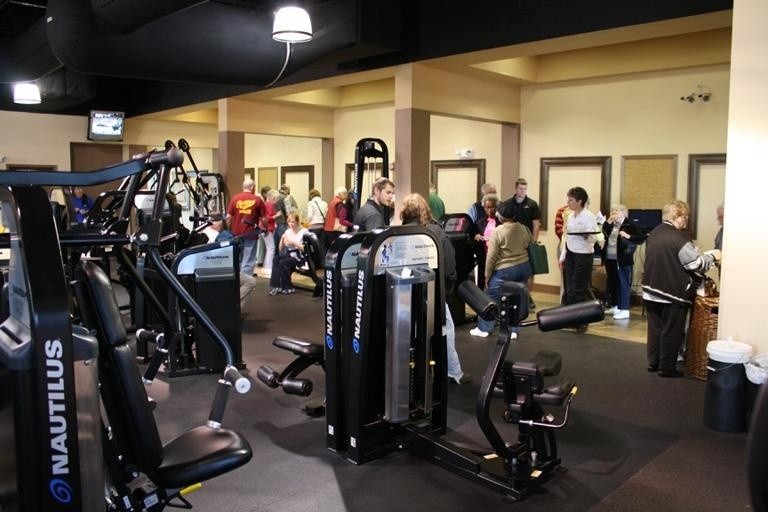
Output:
[613,310,631,319]
[658,368,684,378]
[603,306,620,314]
[469,327,489,338]
[268,286,294,296]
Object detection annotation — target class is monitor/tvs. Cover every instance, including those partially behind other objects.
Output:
[87,110,124,142]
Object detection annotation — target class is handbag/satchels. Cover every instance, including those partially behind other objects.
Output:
[525,225,549,274]
[257,234,267,267]
[333,217,347,231]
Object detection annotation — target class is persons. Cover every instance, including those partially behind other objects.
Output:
[199,179,355,322]
[396,193,457,299]
[555,186,645,320]
[468,178,540,340]
[72,187,93,223]
[426,182,445,221]
[353,177,396,231]
[631,200,724,377]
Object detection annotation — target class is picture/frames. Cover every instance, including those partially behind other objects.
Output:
[687,153,727,242]
[244,167,255,195]
[430,159,486,216]
[345,162,384,195]
[539,156,612,231]
[280,164,315,193]
[620,154,678,211]
[257,166,278,192]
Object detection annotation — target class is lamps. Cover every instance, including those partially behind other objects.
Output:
[1,80,42,105]
[264,5,313,86]
